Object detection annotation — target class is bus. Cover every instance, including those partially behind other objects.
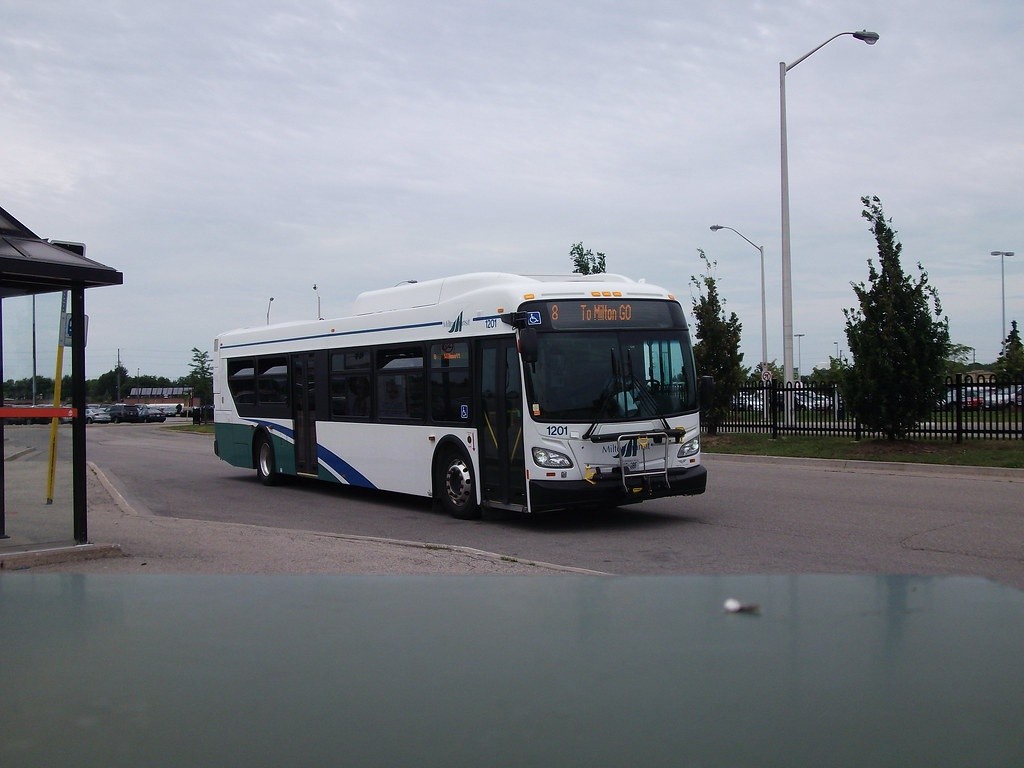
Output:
[212,272,708,520]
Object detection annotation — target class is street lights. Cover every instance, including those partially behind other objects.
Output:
[992,251,1014,368]
[779,29,879,426]
[711,225,769,421]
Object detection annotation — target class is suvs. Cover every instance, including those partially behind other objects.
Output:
[109,405,138,424]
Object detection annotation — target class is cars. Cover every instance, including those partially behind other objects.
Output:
[162,407,176,417]
[732,389,842,410]
[940,385,1024,408]
[180,408,194,416]
[6,404,166,424]
